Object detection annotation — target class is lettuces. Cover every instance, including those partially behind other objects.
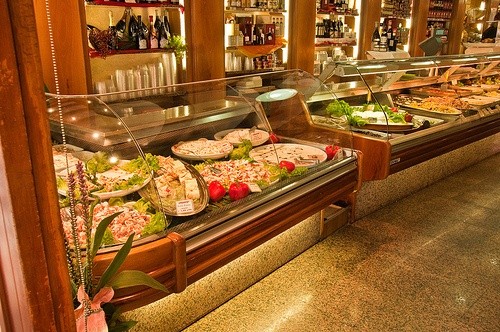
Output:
[326,100,410,127]
[194,138,309,189]
[55,152,167,247]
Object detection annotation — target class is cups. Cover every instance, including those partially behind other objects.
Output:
[225,53,255,71]
[96,53,177,100]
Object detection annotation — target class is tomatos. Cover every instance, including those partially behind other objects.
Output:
[405,114,412,122]
[392,108,398,112]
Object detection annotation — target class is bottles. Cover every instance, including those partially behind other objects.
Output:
[84,0,179,5]
[244,24,264,46]
[316,0,349,39]
[86,10,172,50]
[371,20,405,51]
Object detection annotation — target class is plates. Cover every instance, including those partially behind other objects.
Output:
[51,128,328,255]
[310,78,500,140]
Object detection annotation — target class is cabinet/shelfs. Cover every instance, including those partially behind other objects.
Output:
[32,0,468,108]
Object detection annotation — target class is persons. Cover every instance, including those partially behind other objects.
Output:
[480,11,500,43]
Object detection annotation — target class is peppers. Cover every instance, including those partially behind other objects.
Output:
[326,143,340,160]
[270,134,279,143]
[279,161,295,173]
[229,180,250,201]
[207,180,226,201]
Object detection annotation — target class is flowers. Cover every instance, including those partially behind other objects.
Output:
[58,161,171,332]
